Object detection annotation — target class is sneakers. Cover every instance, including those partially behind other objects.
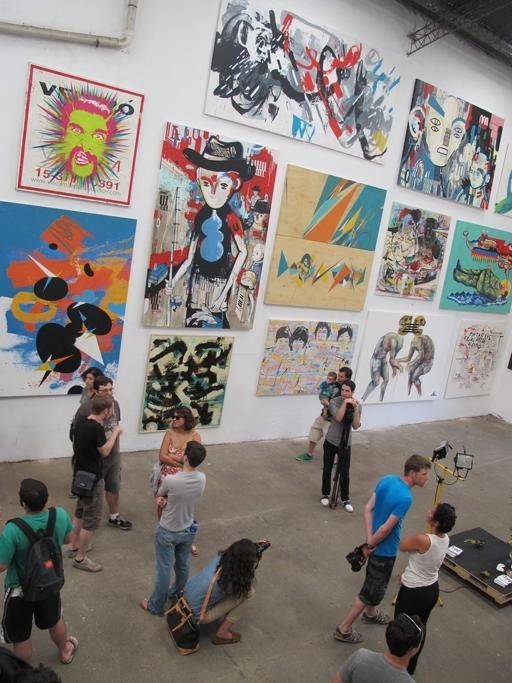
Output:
[212,630,241,645]
[320,461,337,470]
[341,499,354,513]
[191,543,200,556]
[140,597,166,617]
[68,543,93,558]
[320,494,330,507]
[73,557,103,573]
[108,515,132,530]
[360,609,394,624]
[334,626,365,643]
[295,452,314,461]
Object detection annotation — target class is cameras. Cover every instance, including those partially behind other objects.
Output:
[256,541,270,558]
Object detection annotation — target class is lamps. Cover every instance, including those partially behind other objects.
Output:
[429,439,474,479]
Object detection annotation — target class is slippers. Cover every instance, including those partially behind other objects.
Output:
[60,635,79,665]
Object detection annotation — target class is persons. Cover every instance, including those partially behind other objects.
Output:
[336,455,432,642]
[399,504,458,674]
[0,479,78,664]
[89,376,133,531]
[397,316,434,397]
[336,615,423,678]
[68,398,122,572]
[361,316,412,403]
[140,441,206,615]
[453,259,508,299]
[68,367,104,498]
[180,539,270,644]
[157,408,201,556]
[0,646,63,682]
[295,367,353,469]
[321,380,362,514]
[316,372,338,422]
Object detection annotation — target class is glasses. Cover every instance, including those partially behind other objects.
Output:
[172,415,184,420]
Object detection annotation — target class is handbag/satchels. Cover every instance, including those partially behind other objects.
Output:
[165,597,201,655]
[148,461,162,494]
[71,470,98,497]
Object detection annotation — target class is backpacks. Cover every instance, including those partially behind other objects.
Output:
[4,507,65,600]
